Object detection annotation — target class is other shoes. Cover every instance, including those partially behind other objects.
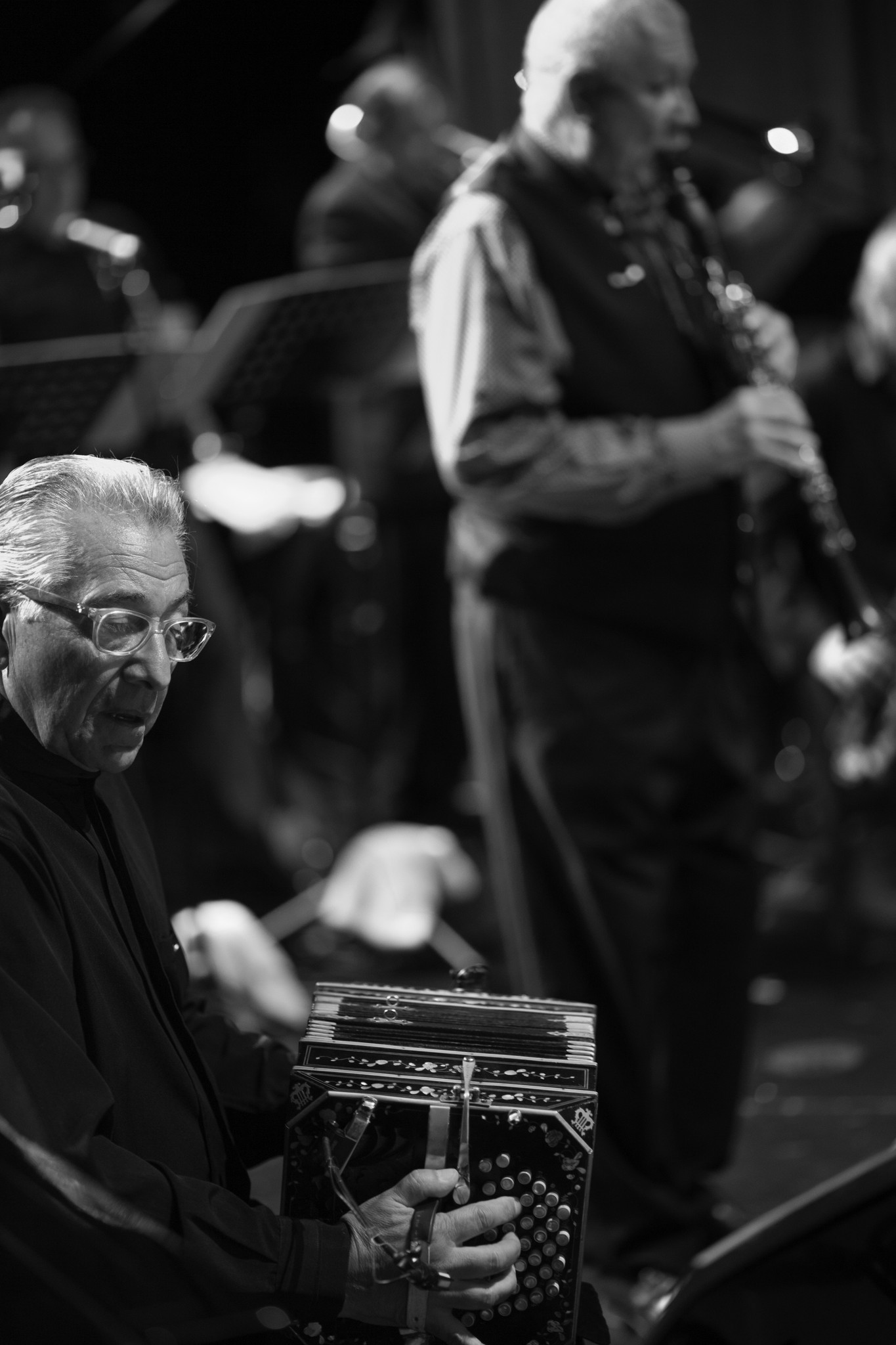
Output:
[608,1272,683,1334]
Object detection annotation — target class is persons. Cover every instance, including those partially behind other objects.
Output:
[407,0,819,1285]
[0,62,522,984]
[0,454,521,1345]
[759,211,896,1027]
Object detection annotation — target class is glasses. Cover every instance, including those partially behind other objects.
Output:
[1,578,216,663]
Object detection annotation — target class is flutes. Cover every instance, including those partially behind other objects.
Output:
[652,145,885,655]
